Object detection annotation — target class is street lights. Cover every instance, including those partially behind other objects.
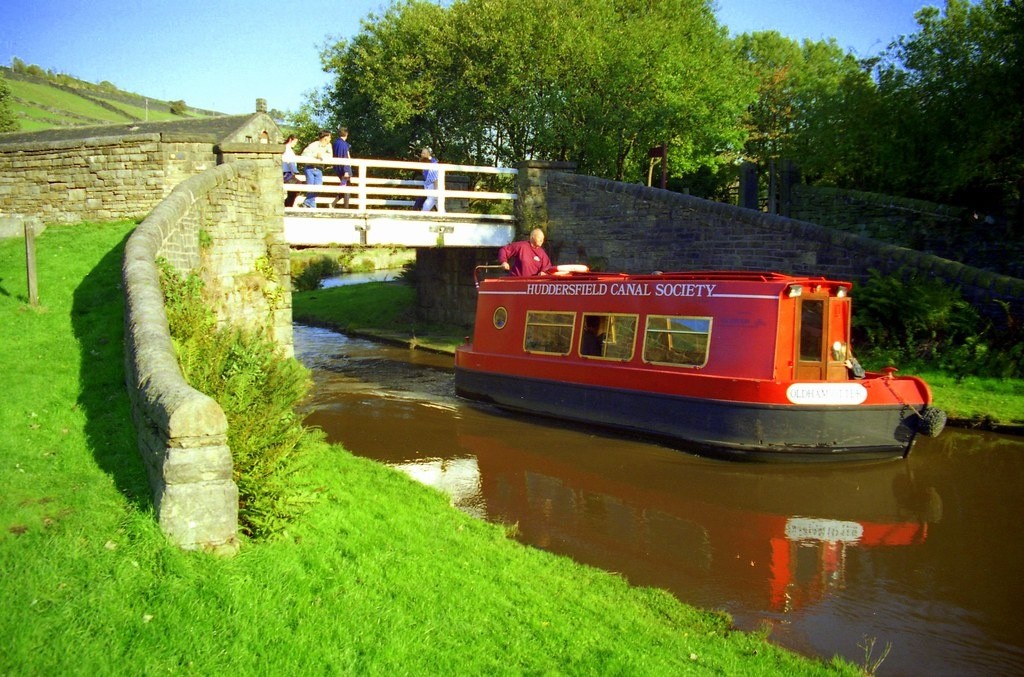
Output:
[648,144,667,189]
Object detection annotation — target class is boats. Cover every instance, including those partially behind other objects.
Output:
[455,265,946,463]
[457,403,942,609]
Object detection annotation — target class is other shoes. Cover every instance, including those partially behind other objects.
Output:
[329,203,335,209]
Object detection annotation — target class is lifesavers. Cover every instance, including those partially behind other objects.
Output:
[547,264,590,275]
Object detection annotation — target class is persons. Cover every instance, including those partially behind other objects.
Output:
[410,145,446,212]
[282,134,300,207]
[328,127,352,208]
[499,229,552,277]
[299,129,333,208]
[581,314,606,357]
